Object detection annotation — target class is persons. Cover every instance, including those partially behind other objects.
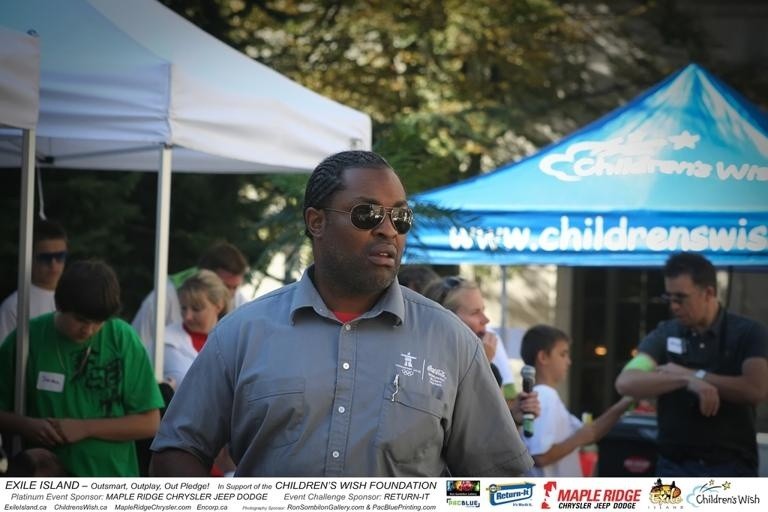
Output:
[1,221,70,345]
[132,239,253,346]
[147,149,537,477]
[396,259,595,477]
[613,250,768,477]
[149,270,240,477]
[0,258,165,476]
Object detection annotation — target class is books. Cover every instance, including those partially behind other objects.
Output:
[594,393,636,443]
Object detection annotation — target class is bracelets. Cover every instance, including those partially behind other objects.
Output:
[621,351,656,373]
[696,367,705,385]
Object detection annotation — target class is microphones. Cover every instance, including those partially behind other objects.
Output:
[520,364,537,438]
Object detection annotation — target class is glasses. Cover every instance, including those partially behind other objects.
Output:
[439,276,464,305]
[32,250,67,262]
[332,203,413,234]
[661,290,698,301]
